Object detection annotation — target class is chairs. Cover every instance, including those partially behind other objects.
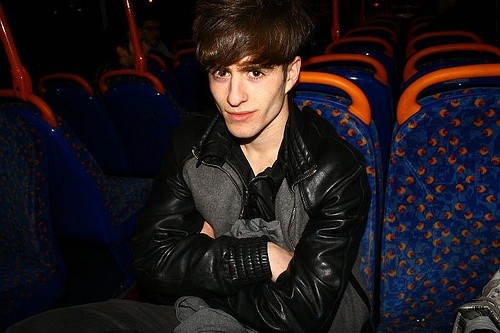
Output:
[0,29,499,333]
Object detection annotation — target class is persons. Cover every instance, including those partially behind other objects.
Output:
[453,267,500,333]
[0,0,371,333]
[128,18,179,57]
